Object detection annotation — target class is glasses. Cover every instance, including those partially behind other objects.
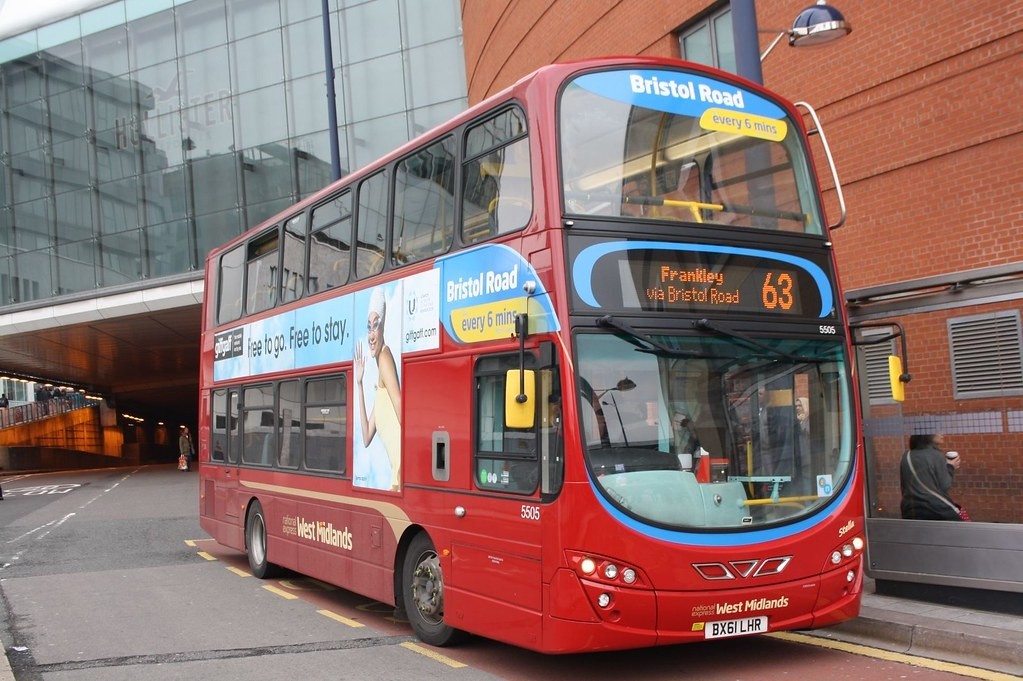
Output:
[366,318,382,334]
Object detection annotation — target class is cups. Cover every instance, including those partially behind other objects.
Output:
[946,451,958,460]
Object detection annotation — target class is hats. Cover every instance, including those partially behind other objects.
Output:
[365,288,387,323]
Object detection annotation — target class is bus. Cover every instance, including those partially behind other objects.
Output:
[198,54,913,655]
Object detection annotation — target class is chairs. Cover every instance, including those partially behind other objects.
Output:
[243,431,344,471]
[486,196,532,237]
[235,252,388,318]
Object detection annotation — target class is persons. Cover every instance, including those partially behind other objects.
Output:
[354,288,401,492]
[596,376,648,443]
[795,397,809,435]
[900,416,966,522]
[179,427,196,472]
[36,385,72,416]
[0,394,9,407]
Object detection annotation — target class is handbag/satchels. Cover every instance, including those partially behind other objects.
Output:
[958,508,972,522]
[177,455,188,470]
